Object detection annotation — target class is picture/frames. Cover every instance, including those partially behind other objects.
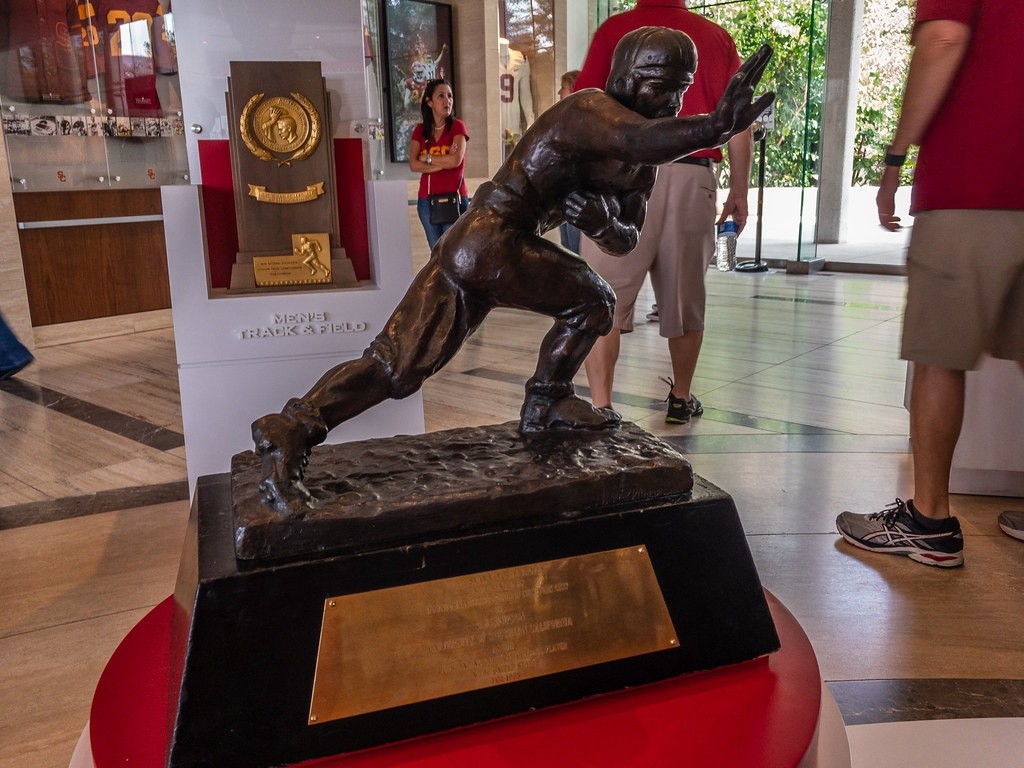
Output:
[377,0,456,163]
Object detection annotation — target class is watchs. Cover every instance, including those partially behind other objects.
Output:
[426,153,432,165]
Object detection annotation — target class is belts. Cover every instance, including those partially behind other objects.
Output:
[673,156,710,167]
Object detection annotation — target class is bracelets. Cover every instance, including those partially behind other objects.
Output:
[883,143,908,167]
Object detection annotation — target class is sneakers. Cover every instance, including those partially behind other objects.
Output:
[997,511,1024,540]
[658,376,703,423]
[835,498,964,568]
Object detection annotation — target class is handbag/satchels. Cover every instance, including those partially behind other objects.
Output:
[428,192,461,226]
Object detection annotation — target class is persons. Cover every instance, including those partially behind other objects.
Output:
[0,315,34,380]
[410,78,469,252]
[405,45,450,110]
[558,70,580,253]
[261,107,297,144]
[498,37,535,161]
[248,25,776,511]
[834,0,1024,567]
[575,0,751,425]
[294,236,330,276]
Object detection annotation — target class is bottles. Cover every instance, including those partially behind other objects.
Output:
[716,202,737,271]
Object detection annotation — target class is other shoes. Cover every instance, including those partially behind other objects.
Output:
[646,311,660,321]
[652,304,657,310]
